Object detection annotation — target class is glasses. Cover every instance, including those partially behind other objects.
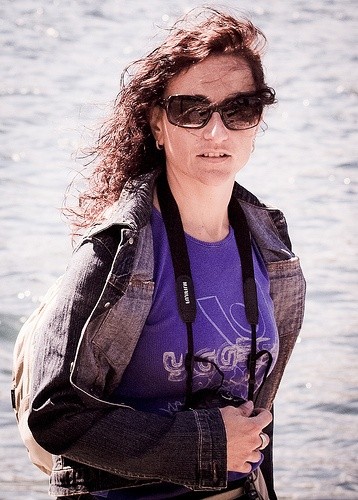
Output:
[157,90,265,131]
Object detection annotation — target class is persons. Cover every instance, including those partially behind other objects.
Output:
[10,3,308,500]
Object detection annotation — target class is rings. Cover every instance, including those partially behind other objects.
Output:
[259,432,267,451]
